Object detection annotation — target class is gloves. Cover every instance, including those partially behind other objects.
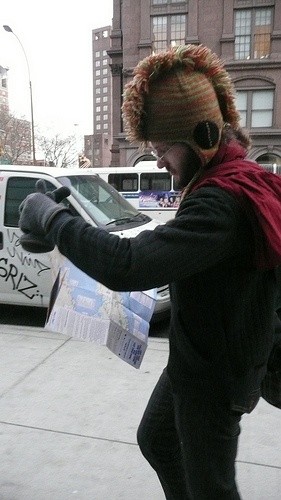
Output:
[18,179,75,253]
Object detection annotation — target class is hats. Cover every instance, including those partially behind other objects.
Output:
[121,44,242,204]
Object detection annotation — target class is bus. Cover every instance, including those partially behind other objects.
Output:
[85,160,183,212]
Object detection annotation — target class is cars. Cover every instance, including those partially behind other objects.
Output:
[0,165,172,315]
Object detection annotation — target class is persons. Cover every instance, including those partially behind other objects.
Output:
[18,43,281,500]
[154,192,177,208]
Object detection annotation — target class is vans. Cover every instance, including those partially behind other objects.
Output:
[2,24,36,164]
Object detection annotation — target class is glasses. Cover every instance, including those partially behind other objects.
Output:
[150,143,179,164]
